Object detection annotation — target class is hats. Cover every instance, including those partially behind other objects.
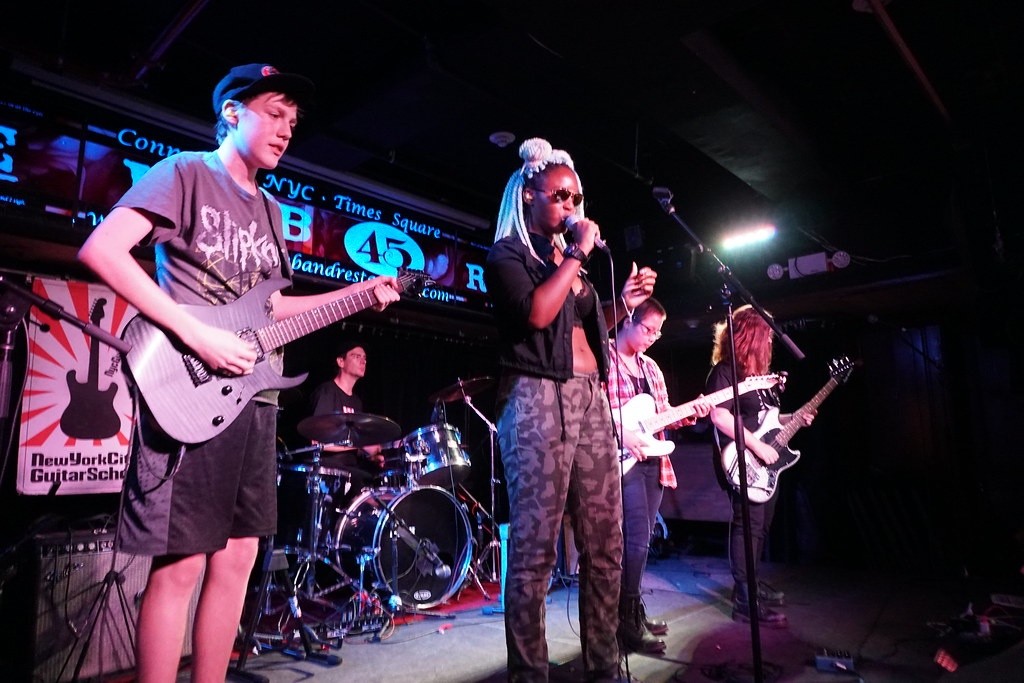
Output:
[212,64,308,115]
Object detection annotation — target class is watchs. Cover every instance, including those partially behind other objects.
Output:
[563,244,588,266]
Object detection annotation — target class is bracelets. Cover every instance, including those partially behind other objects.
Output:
[620,294,634,323]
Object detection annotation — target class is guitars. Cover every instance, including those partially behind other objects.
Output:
[122,267,437,445]
[612,371,790,476]
[715,356,857,506]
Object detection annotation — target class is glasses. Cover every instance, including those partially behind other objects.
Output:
[629,316,662,341]
[527,184,585,208]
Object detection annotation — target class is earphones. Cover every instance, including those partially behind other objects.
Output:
[226,109,236,117]
[526,193,533,199]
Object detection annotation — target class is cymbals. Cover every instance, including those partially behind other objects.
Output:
[297,410,402,445]
[439,374,491,401]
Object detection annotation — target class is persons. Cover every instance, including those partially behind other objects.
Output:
[705,304,819,629]
[311,342,383,459]
[489,137,656,683]
[608,296,716,654]
[78,63,401,683]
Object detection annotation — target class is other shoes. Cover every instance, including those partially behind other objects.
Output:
[582,661,623,683]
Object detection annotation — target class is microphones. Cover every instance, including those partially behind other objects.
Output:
[566,215,611,255]
[435,564,451,578]
[432,399,442,424]
[869,315,909,334]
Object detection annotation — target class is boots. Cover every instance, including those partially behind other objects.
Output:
[731,582,788,629]
[640,603,669,634]
[730,576,787,608]
[615,589,667,651]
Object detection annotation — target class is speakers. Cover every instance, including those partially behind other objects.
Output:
[21,526,154,683]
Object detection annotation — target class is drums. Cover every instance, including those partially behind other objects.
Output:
[404,422,473,484]
[337,487,472,611]
[275,464,350,552]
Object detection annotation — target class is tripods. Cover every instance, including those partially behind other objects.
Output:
[231,421,375,644]
[459,386,502,589]
[372,494,455,642]
